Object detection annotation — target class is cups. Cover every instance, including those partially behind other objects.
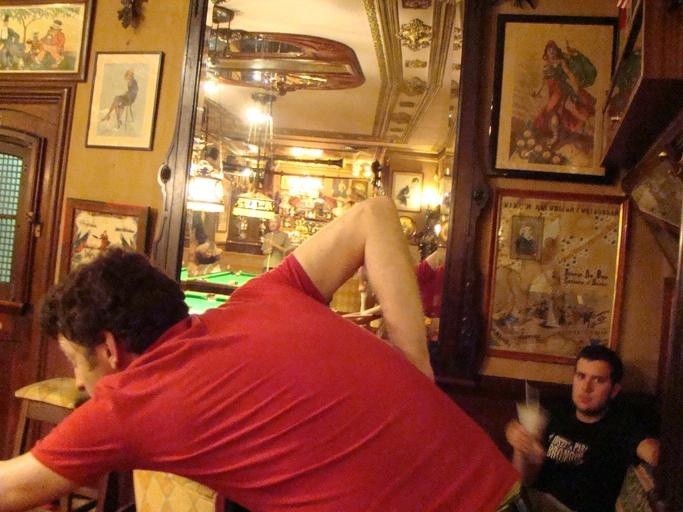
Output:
[516,403,548,441]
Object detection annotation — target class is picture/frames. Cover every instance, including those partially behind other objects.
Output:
[85,51,164,151]
[482,188,631,365]
[0,0,97,82]
[389,168,423,214]
[488,13,618,185]
[58,197,151,284]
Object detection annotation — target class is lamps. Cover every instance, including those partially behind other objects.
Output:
[232,93,277,221]
[186,96,225,212]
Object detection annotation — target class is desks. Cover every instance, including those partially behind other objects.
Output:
[180,268,263,296]
[179,289,233,317]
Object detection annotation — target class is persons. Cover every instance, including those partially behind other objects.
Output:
[504,343,659,512]
[1,14,64,68]
[261,219,292,272]
[99,69,138,129]
[529,39,593,147]
[353,234,445,341]
[0,197,528,512]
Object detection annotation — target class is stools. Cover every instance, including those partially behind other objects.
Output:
[10,377,114,512]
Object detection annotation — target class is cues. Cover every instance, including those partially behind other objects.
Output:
[266,232,273,272]
